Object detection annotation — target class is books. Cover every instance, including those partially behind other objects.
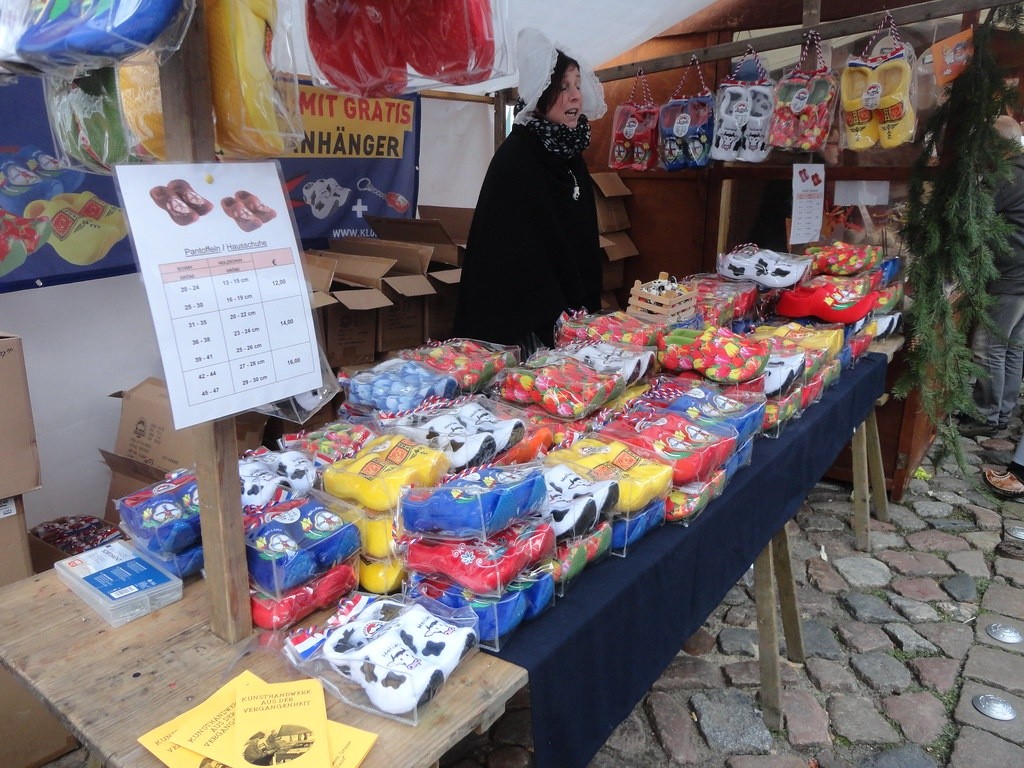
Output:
[136,670,378,768]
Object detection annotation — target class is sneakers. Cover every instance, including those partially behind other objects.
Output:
[717,243,812,288]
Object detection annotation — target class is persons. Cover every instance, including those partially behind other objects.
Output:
[452,27,607,356]
[946,84,1024,497]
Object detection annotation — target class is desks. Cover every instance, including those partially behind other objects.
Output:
[821,289,974,503]
[1,312,904,768]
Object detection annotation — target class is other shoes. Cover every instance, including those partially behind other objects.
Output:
[347,276,913,636]
[805,241,902,287]
[840,62,916,151]
[608,103,659,172]
[770,76,836,152]
[117,417,446,631]
[323,600,476,716]
[658,97,712,171]
[712,86,772,163]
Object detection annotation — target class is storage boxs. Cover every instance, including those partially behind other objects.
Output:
[0,494,34,587]
[263,205,476,447]
[107,377,267,473]
[99,449,167,525]
[0,330,41,499]
[29,516,130,574]
[601,290,620,311]
[590,173,631,234]
[600,231,639,290]
